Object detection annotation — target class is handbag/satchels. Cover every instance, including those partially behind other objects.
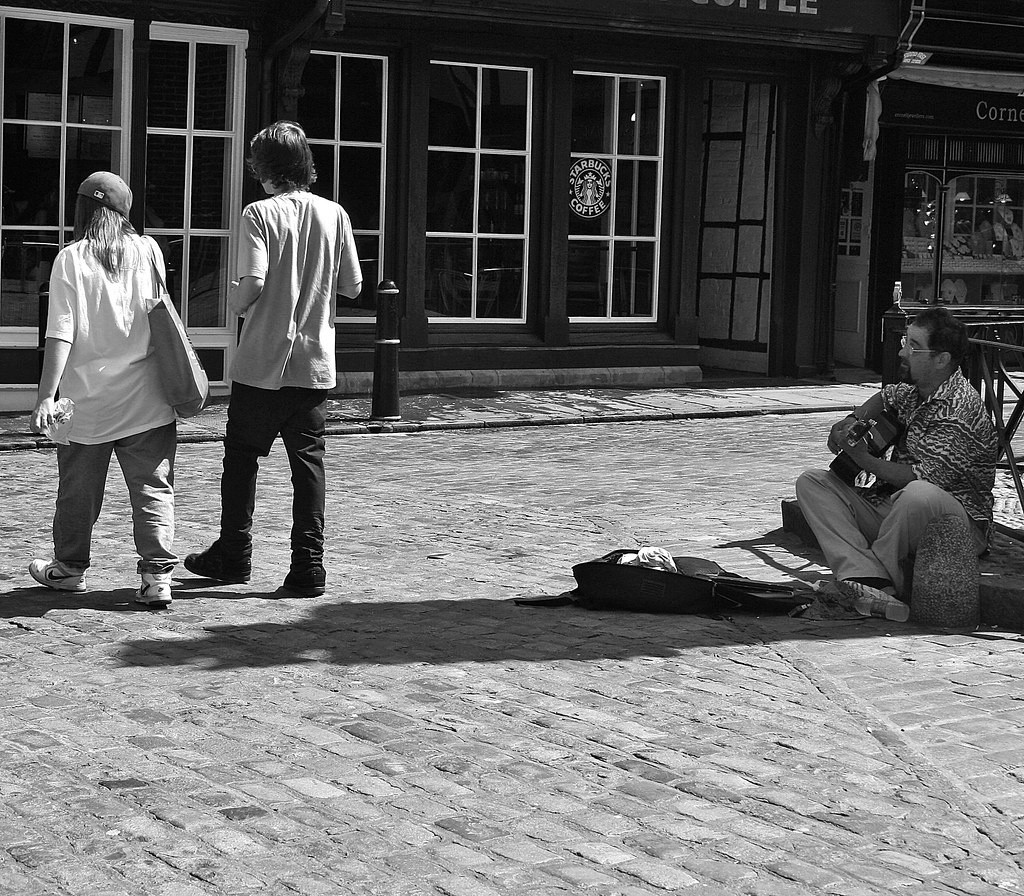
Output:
[146,293,212,419]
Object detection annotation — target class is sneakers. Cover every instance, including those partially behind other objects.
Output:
[28,557,88,592]
[183,539,252,581]
[284,566,327,596]
[134,572,174,606]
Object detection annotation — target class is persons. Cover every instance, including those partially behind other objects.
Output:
[28,169,184,607]
[184,122,366,598]
[794,305,1000,617]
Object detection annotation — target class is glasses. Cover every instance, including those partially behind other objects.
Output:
[900,335,938,355]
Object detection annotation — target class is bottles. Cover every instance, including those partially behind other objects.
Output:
[851,596,909,622]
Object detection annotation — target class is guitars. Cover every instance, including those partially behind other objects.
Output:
[829,411,905,488]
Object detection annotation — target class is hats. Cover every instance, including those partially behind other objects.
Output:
[77,171,133,221]
